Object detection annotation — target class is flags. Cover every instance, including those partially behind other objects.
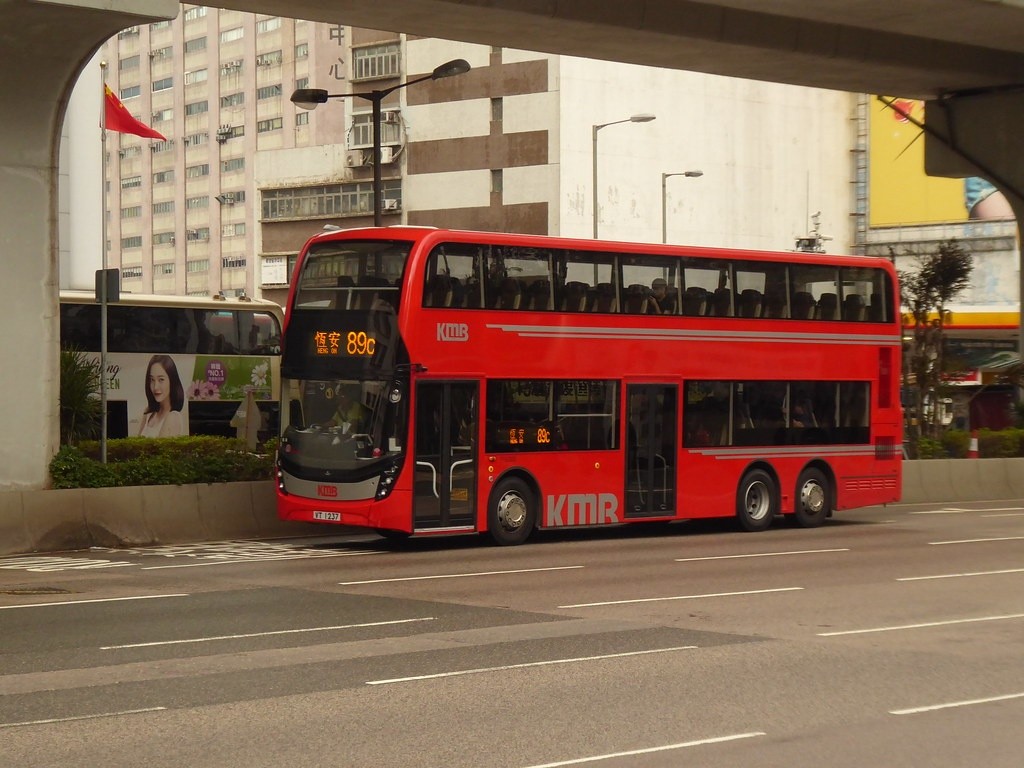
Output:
[105,84,166,141]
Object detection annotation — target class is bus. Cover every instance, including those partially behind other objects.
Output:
[272,223,904,544]
[57,288,292,457]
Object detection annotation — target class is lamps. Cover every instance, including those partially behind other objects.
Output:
[215,194,233,204]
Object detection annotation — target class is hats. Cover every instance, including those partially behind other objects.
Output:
[652,278,667,288]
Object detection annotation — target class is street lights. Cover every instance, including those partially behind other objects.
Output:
[288,58,472,270]
[660,171,706,284]
[591,113,658,289]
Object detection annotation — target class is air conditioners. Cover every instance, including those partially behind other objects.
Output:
[224,192,234,204]
[170,236,175,243]
[345,150,364,166]
[189,229,197,234]
[216,128,232,141]
[256,50,307,66]
[120,25,138,34]
[148,49,164,56]
[380,199,397,210]
[379,147,393,164]
[220,61,240,69]
[380,112,395,122]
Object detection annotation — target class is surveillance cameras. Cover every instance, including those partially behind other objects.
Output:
[811,211,820,217]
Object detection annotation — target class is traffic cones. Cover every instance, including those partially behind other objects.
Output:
[968,431,980,460]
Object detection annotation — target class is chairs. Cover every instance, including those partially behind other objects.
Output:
[739,398,852,428]
[336,275,867,322]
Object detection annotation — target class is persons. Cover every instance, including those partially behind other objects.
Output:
[647,278,673,314]
[783,400,814,427]
[139,355,184,436]
[321,384,367,436]
